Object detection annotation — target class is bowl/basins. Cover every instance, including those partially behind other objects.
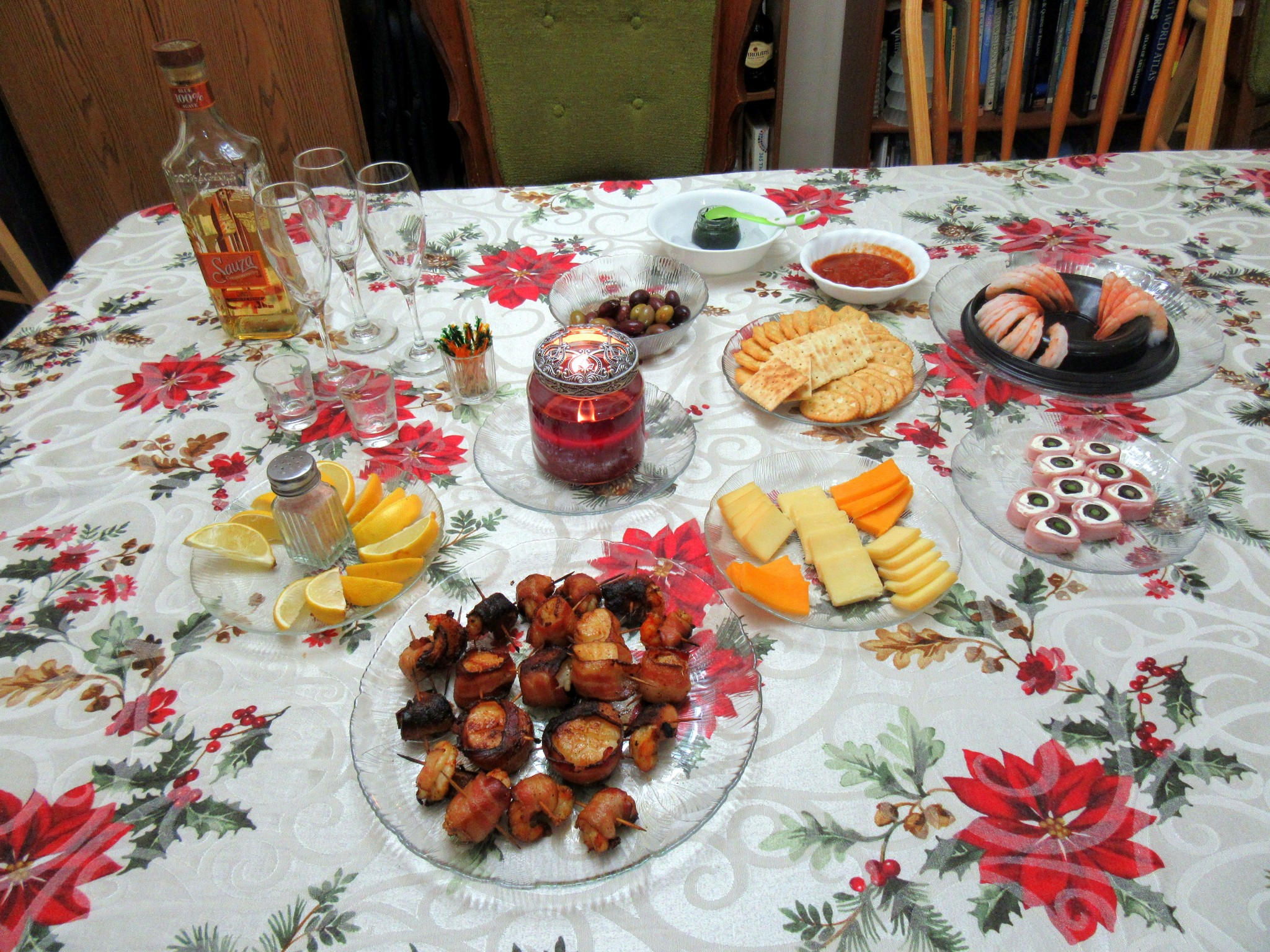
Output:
[549,253,709,361]
[798,228,931,305]
[648,186,786,273]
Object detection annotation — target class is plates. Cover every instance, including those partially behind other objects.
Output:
[720,306,928,429]
[350,535,764,890]
[950,410,1209,573]
[472,380,697,515]
[190,458,444,635]
[704,448,962,633]
[926,248,1228,403]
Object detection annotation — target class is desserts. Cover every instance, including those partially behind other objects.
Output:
[1006,433,1159,552]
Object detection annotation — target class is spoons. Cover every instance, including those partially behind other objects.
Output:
[704,206,821,227]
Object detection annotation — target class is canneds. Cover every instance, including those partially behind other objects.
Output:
[526,324,645,485]
[691,205,741,250]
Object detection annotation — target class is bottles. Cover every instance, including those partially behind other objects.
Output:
[267,450,355,572]
[527,323,646,485]
[744,0,775,93]
[692,205,741,250]
[155,37,308,340]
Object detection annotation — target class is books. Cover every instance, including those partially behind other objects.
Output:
[743,111,770,173]
[865,133,1021,170]
[869,1,1192,130]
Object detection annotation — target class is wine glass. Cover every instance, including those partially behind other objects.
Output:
[253,181,366,402]
[293,147,399,354]
[355,161,448,377]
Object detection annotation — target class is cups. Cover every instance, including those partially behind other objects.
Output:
[338,368,400,449]
[253,353,319,432]
[439,328,498,405]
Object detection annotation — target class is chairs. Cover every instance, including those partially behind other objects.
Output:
[902,1,1232,167]
[428,0,756,194]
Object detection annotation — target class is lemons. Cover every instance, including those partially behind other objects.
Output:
[185,460,439,631]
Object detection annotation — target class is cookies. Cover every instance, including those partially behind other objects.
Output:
[732,304,916,422]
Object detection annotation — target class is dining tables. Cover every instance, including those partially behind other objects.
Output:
[0,149,1269,952]
